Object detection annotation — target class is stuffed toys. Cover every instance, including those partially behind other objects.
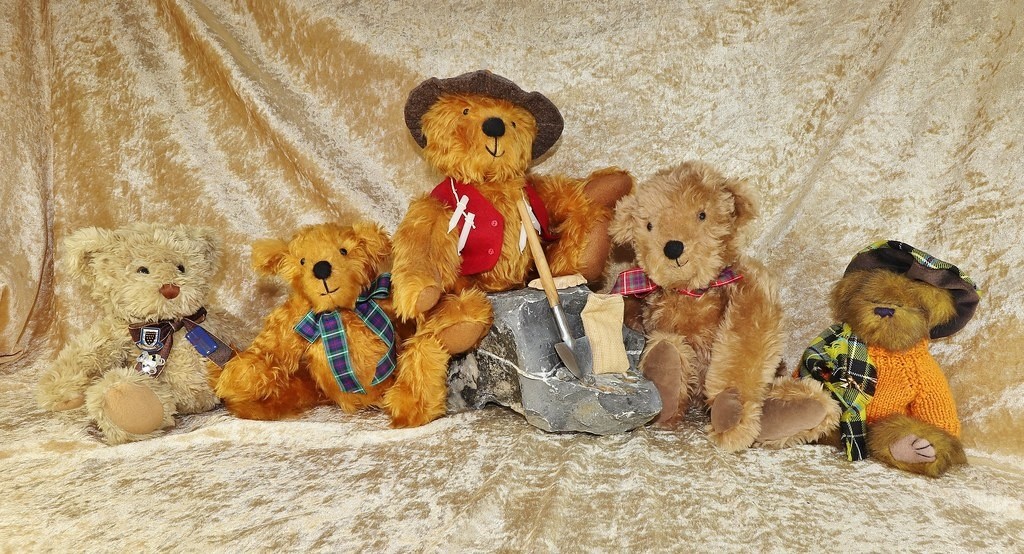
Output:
[603,158,842,447]
[219,213,499,427]
[43,217,242,443]
[775,238,981,477]
[382,66,634,356]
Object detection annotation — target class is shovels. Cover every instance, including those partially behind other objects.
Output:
[515,200,594,381]
